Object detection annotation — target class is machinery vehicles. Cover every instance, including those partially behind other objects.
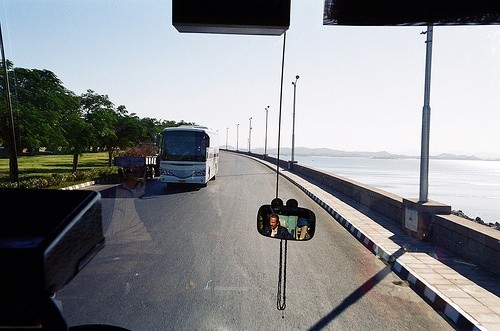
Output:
[113,143,158,181]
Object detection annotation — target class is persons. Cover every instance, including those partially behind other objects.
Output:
[262,214,297,240]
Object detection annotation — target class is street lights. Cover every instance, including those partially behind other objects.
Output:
[291,75,300,161]
[226,127,229,149]
[265,106,269,154]
[249,116,252,151]
[236,124,239,150]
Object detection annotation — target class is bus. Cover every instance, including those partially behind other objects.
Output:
[155,125,219,187]
